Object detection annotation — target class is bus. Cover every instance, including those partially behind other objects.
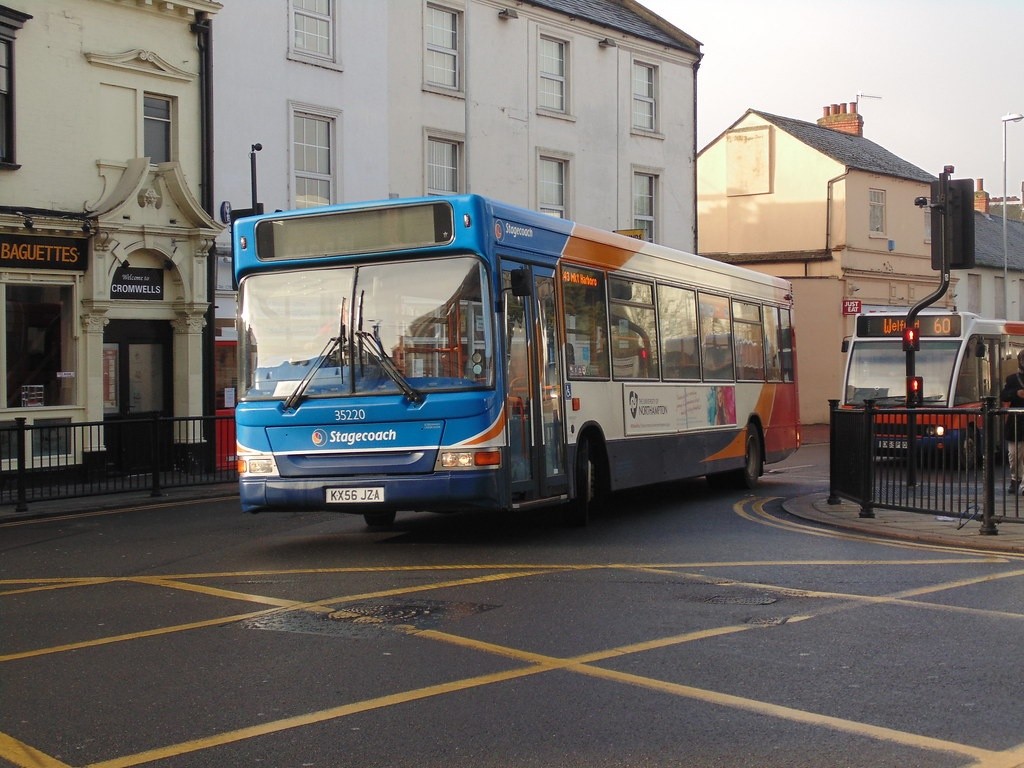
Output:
[231,194,801,531]
[840,307,1023,472]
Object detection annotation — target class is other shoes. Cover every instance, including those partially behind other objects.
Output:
[1007,479,1022,493]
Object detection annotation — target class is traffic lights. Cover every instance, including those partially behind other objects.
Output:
[901,327,921,351]
[905,376,924,408]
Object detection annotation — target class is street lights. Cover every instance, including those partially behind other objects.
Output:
[1002,112,1024,320]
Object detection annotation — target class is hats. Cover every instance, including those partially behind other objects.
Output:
[1017,350,1024,370]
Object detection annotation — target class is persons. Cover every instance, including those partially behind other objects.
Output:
[1000,350,1024,495]
[304,310,342,358]
